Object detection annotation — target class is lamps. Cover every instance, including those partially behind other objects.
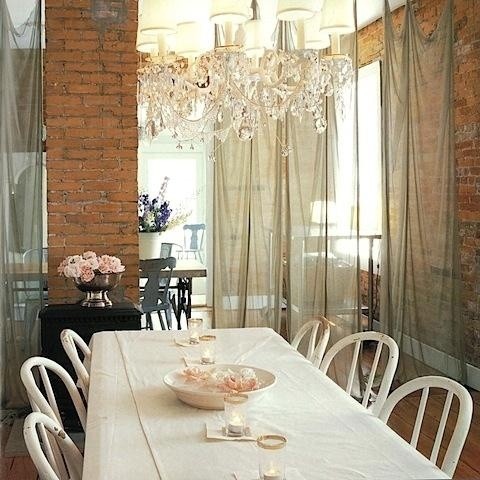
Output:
[135,0,355,162]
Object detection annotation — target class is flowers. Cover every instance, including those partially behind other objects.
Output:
[137,175,194,233]
[57,250,125,283]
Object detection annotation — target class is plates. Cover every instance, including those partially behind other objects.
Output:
[175,334,201,346]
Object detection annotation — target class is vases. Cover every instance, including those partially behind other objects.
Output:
[71,272,122,307]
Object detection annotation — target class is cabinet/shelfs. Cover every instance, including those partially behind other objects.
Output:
[38,304,143,432]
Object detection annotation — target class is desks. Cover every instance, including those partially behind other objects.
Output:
[261,225,381,336]
[75,327,452,480]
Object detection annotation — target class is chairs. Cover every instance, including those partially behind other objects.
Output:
[175,223,206,265]
[139,256,176,330]
[60,329,91,391]
[378,376,474,477]
[19,356,86,480]
[23,411,83,480]
[320,331,399,416]
[290,316,330,368]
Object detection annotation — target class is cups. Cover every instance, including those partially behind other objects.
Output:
[189,318,217,364]
[257,434,287,480]
[224,393,248,437]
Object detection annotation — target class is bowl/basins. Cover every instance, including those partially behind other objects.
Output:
[163,363,278,410]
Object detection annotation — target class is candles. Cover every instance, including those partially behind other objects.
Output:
[201,348,214,362]
[191,332,199,342]
[264,461,281,480]
[228,410,243,433]
